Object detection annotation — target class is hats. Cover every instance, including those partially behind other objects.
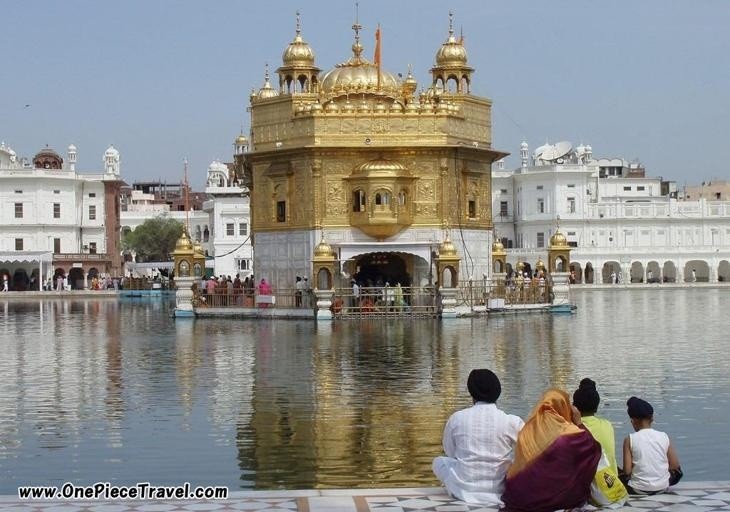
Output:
[466,368,502,403]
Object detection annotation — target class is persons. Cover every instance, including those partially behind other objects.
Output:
[692,269,697,283]
[611,269,633,284]
[201,274,255,307]
[301,275,311,308]
[43,275,67,293]
[573,378,629,509]
[2,279,8,293]
[648,270,653,284]
[617,397,683,497]
[294,276,303,307]
[350,277,403,315]
[89,273,168,291]
[504,269,545,304]
[431,369,525,507]
[499,387,602,512]
[570,270,577,284]
[257,278,274,308]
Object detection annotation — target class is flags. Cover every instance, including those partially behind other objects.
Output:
[374,26,380,64]
